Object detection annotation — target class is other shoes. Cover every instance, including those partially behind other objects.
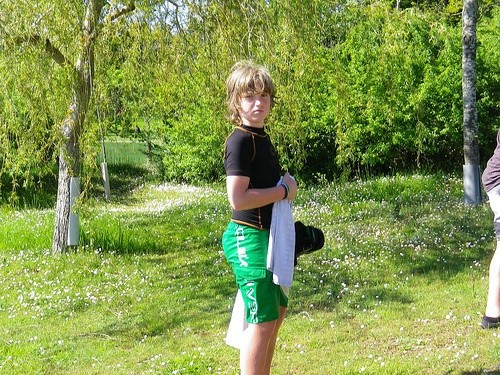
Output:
[481,314,500,329]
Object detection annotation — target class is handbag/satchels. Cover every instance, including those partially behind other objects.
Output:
[226,288,249,349]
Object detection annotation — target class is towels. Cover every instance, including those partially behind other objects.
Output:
[265,173,301,288]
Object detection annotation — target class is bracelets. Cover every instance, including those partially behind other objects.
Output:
[282,183,289,198]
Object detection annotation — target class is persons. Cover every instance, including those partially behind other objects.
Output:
[482,129,500,328]
[222,61,297,375]
